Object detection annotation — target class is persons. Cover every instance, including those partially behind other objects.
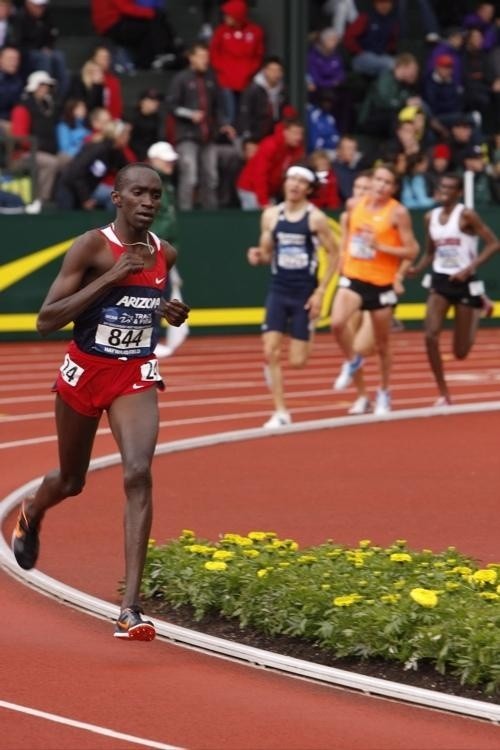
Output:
[402,174,496,414]
[245,163,342,436]
[331,169,416,393]
[10,162,187,641]
[1,0,499,218]
[332,163,419,422]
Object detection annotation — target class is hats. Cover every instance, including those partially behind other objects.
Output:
[148,141,179,162]
[25,69,55,91]
[430,145,451,160]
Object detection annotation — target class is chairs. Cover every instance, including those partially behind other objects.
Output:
[51,0,222,124]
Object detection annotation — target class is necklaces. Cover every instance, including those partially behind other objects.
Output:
[111,222,155,256]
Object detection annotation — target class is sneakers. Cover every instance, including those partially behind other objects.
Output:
[12,495,39,569]
[435,394,450,407]
[113,606,156,641]
[375,386,392,417]
[347,395,371,415]
[264,410,294,427]
[334,355,366,389]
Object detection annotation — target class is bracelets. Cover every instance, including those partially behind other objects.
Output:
[396,273,405,282]
